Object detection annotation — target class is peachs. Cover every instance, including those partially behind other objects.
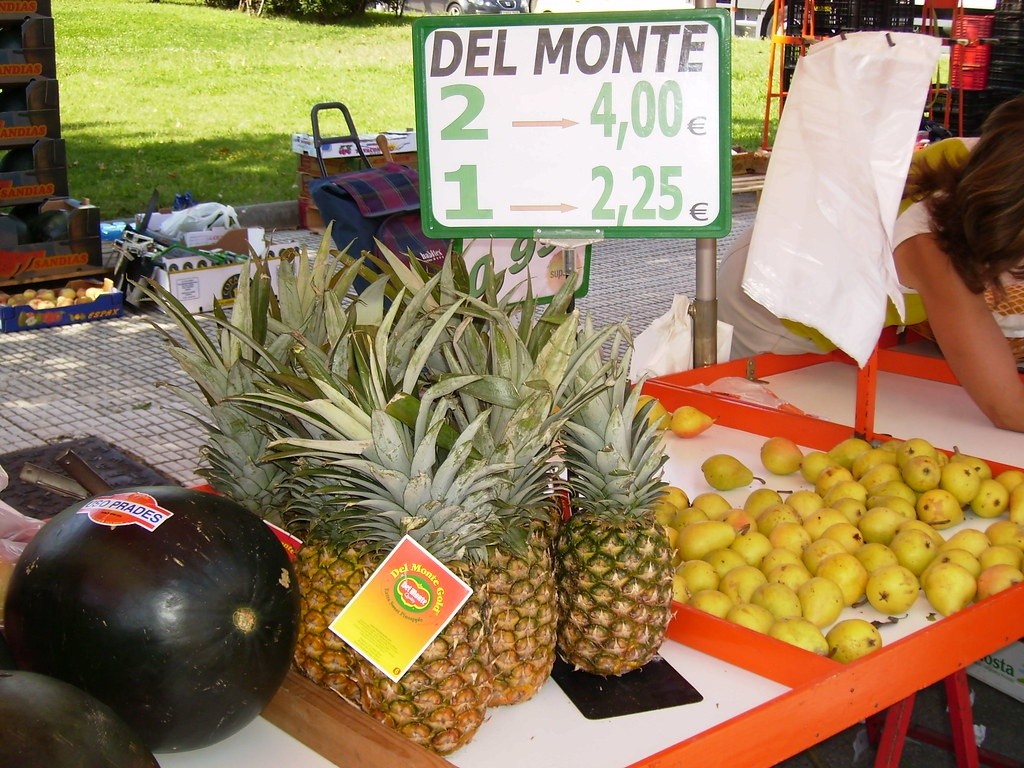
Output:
[0,285,109,310]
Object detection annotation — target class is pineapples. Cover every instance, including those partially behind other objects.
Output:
[128,234,677,754]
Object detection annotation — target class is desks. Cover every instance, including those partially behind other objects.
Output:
[156,316,1024,768]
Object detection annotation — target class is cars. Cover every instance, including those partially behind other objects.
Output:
[401,0,531,16]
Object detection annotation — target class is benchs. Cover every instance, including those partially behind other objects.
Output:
[732,175,766,214]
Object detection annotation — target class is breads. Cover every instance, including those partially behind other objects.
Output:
[981,283,1024,366]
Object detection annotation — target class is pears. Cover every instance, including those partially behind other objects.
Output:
[634,395,1024,663]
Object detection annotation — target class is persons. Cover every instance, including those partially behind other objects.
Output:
[716,95,1024,432]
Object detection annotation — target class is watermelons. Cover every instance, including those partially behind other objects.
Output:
[0,21,73,244]
[0,485,299,768]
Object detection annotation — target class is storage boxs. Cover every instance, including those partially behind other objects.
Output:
[0,0,304,334]
[731,144,770,175]
[292,127,420,234]
[781,0,1024,139]
